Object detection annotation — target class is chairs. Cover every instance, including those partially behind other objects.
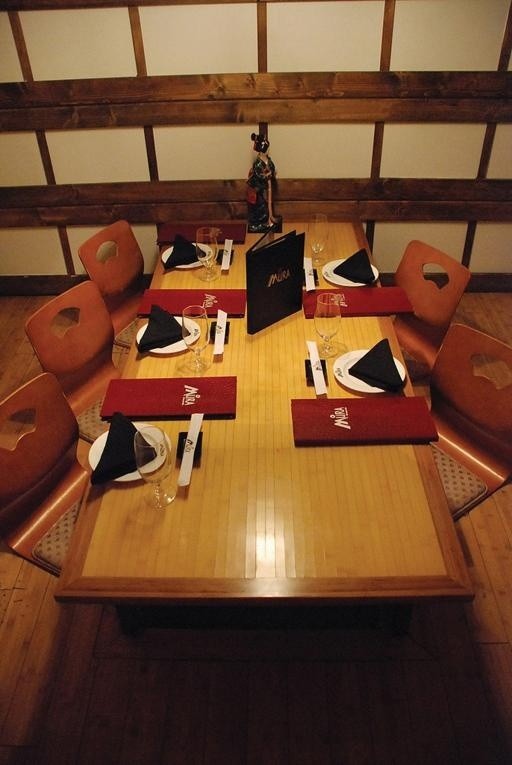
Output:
[428,323,512,521]
[0,372,92,577]
[78,219,146,347]
[24,281,123,444]
[391,240,473,383]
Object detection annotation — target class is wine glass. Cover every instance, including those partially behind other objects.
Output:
[181,305,213,372]
[196,227,219,282]
[313,293,343,358]
[133,426,178,512]
[307,213,329,267]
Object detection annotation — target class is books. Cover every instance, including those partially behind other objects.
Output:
[245,224,305,335]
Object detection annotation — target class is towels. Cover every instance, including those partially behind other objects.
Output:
[163,233,206,272]
[90,410,158,485]
[334,248,375,285]
[348,338,404,394]
[138,303,190,353]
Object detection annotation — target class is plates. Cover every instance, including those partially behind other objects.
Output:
[333,349,406,394]
[87,422,172,483]
[161,242,213,269]
[135,316,200,355]
[322,258,380,289]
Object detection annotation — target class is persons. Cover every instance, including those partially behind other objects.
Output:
[247,133,279,229]
